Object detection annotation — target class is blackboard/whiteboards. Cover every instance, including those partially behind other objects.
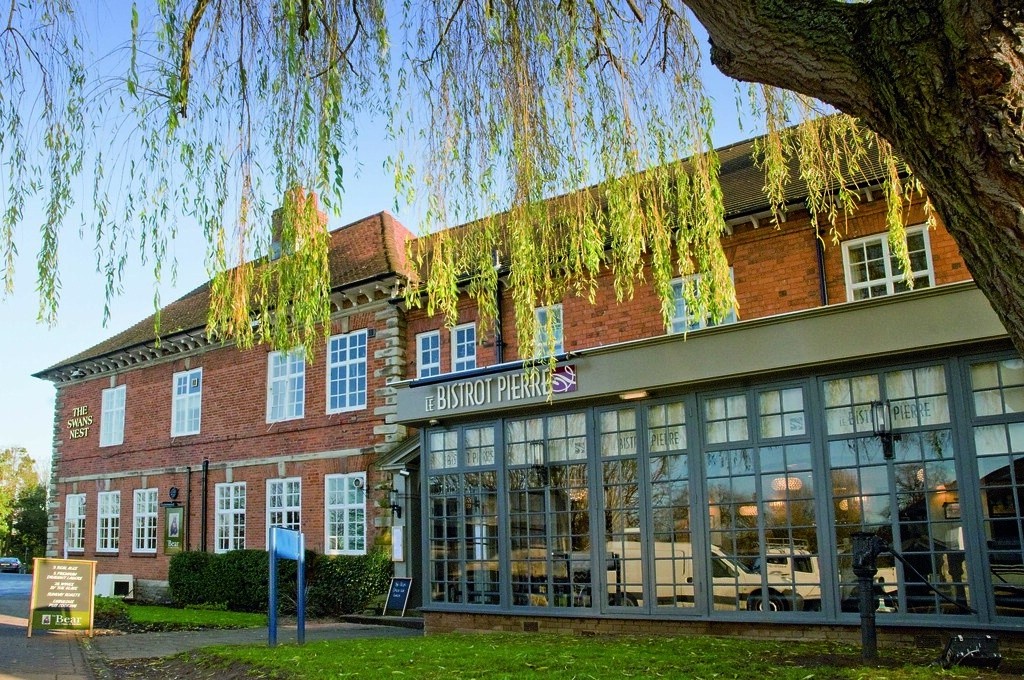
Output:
[384,577,413,609]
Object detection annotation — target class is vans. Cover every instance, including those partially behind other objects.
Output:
[757,549,821,597]
[603,540,804,614]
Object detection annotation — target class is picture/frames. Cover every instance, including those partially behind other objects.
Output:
[943,501,960,520]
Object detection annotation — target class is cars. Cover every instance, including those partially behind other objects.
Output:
[0,558,23,575]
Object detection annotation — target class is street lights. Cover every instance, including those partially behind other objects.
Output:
[872,398,907,613]
